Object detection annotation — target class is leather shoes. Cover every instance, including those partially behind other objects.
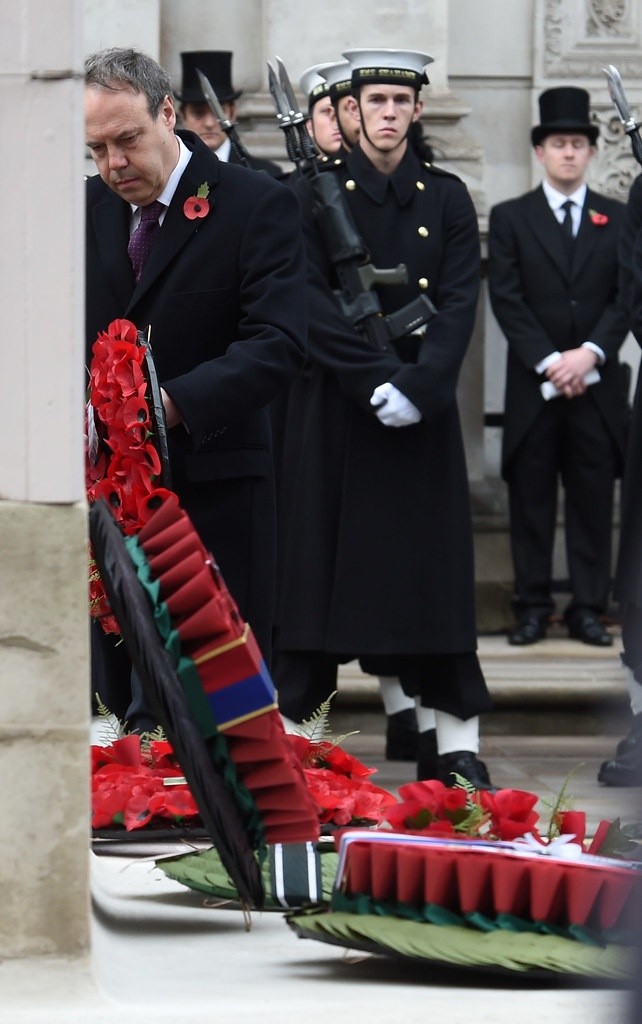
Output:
[438,751,501,790]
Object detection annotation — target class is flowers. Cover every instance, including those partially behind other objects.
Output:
[83,320,169,645]
[90,687,642,936]
[589,210,608,224]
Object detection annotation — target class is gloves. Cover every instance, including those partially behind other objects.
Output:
[369,381,422,428]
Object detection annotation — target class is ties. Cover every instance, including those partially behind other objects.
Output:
[126,200,165,286]
[560,201,576,238]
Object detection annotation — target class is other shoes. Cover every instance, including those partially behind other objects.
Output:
[508,613,551,645]
[417,729,438,781]
[386,708,420,761]
[568,615,612,646]
[598,732,642,785]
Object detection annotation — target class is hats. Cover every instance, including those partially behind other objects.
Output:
[531,86,599,147]
[317,60,352,102]
[300,62,329,114]
[342,48,434,90]
[172,51,243,103]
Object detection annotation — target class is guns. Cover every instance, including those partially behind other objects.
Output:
[264,59,307,187]
[194,66,267,175]
[602,61,642,168]
[270,54,440,374]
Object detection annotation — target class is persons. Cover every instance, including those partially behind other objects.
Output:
[91,47,501,795]
[85,47,312,740]
[486,86,632,647]
[598,173,642,785]
